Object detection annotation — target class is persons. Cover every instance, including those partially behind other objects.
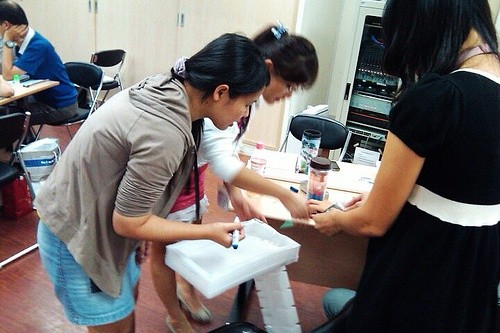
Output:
[0,0,78,164]
[310,0,500,333]
[146,24,319,333]
[33,32,270,333]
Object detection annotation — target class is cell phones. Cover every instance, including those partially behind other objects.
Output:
[13,75,30,84]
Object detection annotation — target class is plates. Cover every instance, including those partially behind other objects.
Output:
[300,180,308,193]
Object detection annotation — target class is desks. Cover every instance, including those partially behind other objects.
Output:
[227,149,378,333]
[0,82,59,105]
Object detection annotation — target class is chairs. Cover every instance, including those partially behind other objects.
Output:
[279,114,352,162]
[91,49,126,107]
[0,112,36,199]
[31,62,103,142]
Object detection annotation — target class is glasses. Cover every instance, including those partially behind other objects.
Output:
[287,82,302,92]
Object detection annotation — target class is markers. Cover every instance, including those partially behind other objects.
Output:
[232,215,240,250]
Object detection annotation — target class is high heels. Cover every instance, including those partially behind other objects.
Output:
[175,283,212,325]
[165,315,177,333]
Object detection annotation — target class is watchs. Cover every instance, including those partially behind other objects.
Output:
[4,41,18,48]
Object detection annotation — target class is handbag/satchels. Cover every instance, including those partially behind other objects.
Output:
[18,137,63,196]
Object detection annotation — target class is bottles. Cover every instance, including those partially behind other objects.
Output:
[246,142,267,198]
[14,75,21,92]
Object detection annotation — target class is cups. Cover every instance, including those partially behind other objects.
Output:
[297,130,322,175]
[307,157,332,201]
